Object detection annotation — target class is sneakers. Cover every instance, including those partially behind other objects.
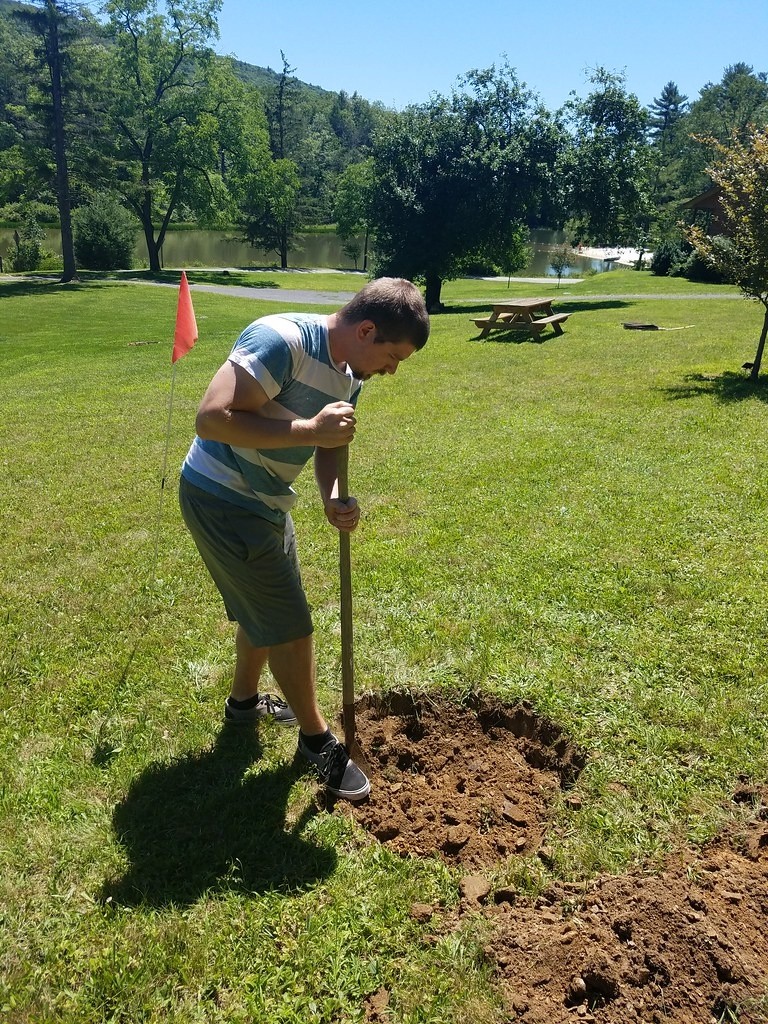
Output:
[297,726,371,801]
[224,694,298,725]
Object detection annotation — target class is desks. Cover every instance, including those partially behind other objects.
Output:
[477,298,563,344]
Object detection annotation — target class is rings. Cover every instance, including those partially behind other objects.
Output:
[352,519,355,527]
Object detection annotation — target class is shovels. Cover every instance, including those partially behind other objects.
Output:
[331,447,377,783]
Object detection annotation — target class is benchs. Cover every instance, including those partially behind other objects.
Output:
[469,313,572,332]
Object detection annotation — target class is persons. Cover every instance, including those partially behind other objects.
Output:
[179,277,430,800]
